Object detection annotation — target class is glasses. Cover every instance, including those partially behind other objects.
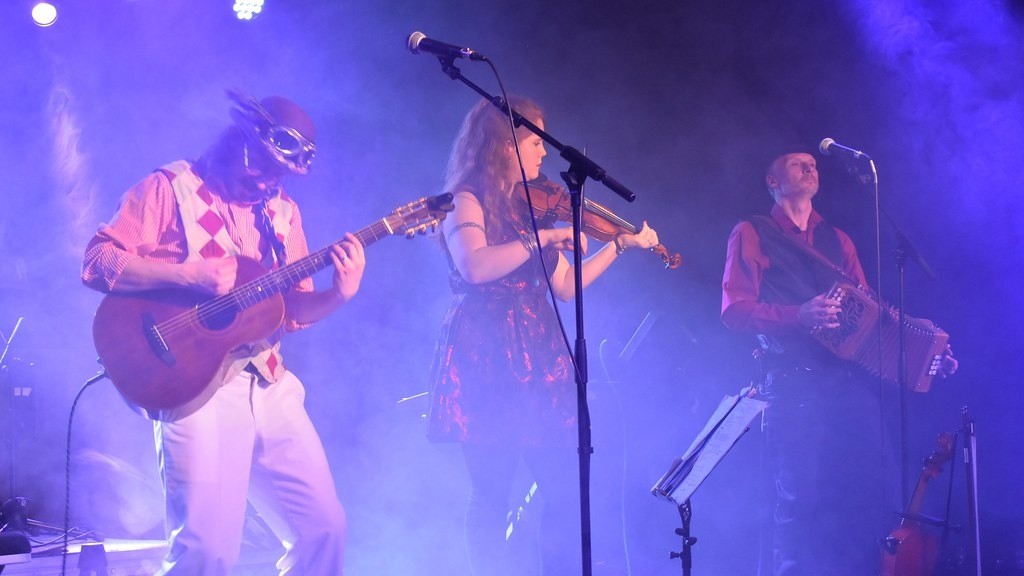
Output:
[266,126,317,169]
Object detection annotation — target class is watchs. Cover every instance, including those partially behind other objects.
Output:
[613,237,624,256]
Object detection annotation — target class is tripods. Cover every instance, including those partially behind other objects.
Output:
[0,319,104,541]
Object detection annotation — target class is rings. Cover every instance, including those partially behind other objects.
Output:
[340,255,348,261]
[648,240,652,246]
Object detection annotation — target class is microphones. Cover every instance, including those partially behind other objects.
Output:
[406,32,489,62]
[818,138,871,161]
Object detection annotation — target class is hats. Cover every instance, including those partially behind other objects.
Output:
[230,85,316,177]
[766,140,815,168]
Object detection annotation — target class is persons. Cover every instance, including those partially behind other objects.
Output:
[720,130,958,576]
[81,90,366,576]
[428,95,658,576]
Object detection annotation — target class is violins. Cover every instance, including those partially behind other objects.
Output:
[513,172,683,271]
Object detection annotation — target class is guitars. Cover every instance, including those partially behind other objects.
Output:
[89,188,457,422]
[881,432,956,575]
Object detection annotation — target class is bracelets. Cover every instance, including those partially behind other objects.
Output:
[519,234,539,259]
